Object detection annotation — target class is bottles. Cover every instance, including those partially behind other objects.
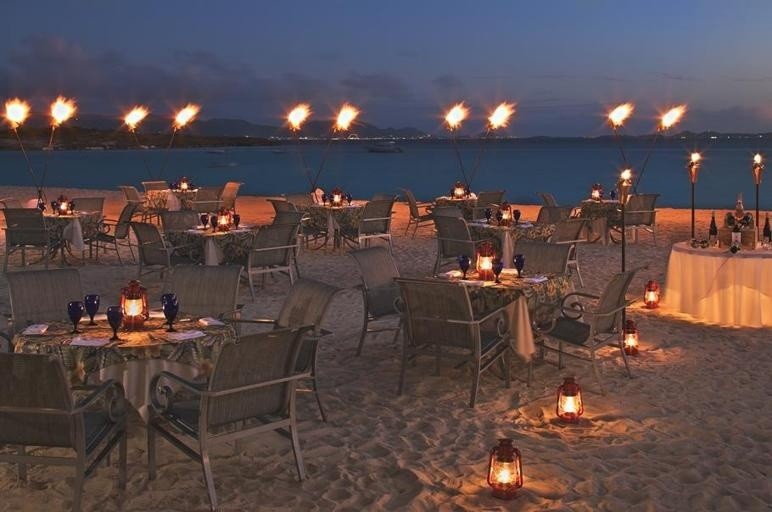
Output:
[763,213,772,242]
[731,224,742,244]
[709,211,717,243]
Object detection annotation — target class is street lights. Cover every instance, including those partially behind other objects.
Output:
[688,153,699,239]
[751,152,765,243]
[157,102,200,181]
[285,102,315,193]
[442,100,470,197]
[631,104,688,195]
[121,104,154,182]
[2,98,43,202]
[310,103,361,192]
[42,94,80,195]
[617,168,629,272]
[468,99,517,194]
[607,101,637,194]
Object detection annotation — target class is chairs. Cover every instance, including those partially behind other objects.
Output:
[119,185,160,225]
[283,192,316,247]
[607,193,659,246]
[142,180,168,189]
[431,206,465,265]
[404,189,436,242]
[1,352,127,512]
[391,276,519,409]
[433,216,496,270]
[343,200,394,255]
[515,220,586,289]
[6,267,85,339]
[536,206,571,225]
[87,202,137,267]
[526,271,636,395]
[2,196,30,208]
[535,206,565,224]
[193,182,243,217]
[160,210,199,261]
[171,262,246,319]
[344,246,402,355]
[367,194,400,249]
[242,222,298,303]
[474,190,505,221]
[0,207,52,274]
[71,196,107,255]
[536,192,586,239]
[128,221,173,281]
[147,328,309,512]
[233,276,346,424]
[431,215,492,276]
[265,198,311,249]
[513,239,574,282]
[267,210,303,277]
[356,200,394,250]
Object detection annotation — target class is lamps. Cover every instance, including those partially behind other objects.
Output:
[643,278,662,309]
[498,200,513,225]
[620,319,641,356]
[487,437,524,501]
[331,185,344,208]
[477,241,497,280]
[217,206,231,230]
[556,376,584,424]
[57,195,68,216]
[116,279,152,332]
[178,176,190,196]
[453,181,466,198]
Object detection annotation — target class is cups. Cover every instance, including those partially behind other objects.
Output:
[688,229,700,249]
[698,230,711,249]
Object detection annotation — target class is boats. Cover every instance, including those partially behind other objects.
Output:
[369,140,401,152]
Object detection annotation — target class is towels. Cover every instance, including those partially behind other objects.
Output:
[148,311,165,319]
[474,223,490,227]
[168,328,207,340]
[462,279,498,287]
[199,317,226,326]
[519,223,533,228]
[70,335,110,346]
[438,270,462,276]
[22,324,49,333]
[476,218,491,222]
[79,315,109,320]
[524,275,548,282]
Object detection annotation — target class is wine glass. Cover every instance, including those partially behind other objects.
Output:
[232,213,243,230]
[66,299,85,336]
[343,189,353,206]
[450,190,455,199]
[495,210,504,227]
[160,292,181,333]
[38,202,46,212]
[321,191,335,207]
[457,253,473,281]
[513,209,522,227]
[188,179,193,190]
[69,200,75,216]
[484,207,494,224]
[512,253,526,280]
[51,200,57,216]
[210,214,220,233]
[83,292,102,327]
[200,213,211,232]
[608,188,620,205]
[106,304,124,342]
[489,255,505,284]
[169,180,179,191]
